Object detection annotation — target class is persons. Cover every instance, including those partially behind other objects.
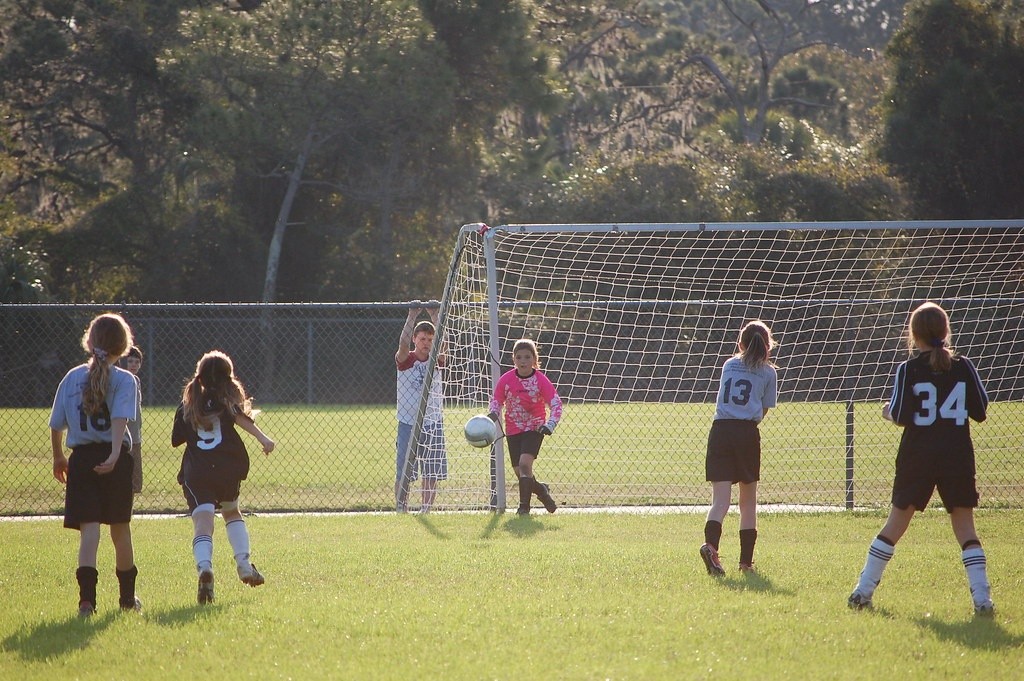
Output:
[48,315,143,617]
[699,321,777,577]
[394,300,449,515]
[172,351,275,606]
[120,347,144,493]
[487,339,562,516]
[846,301,994,617]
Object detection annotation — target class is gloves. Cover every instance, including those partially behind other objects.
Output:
[486,413,498,422]
[538,425,551,436]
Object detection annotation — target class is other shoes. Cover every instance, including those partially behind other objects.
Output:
[78,601,97,619]
[516,503,531,516]
[119,596,141,615]
[536,483,555,513]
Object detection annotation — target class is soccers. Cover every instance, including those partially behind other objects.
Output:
[464,414,497,449]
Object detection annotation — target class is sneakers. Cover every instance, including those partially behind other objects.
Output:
[974,600,993,620]
[700,543,725,577]
[737,563,754,574]
[846,590,873,612]
[237,564,265,587]
[197,570,214,604]
